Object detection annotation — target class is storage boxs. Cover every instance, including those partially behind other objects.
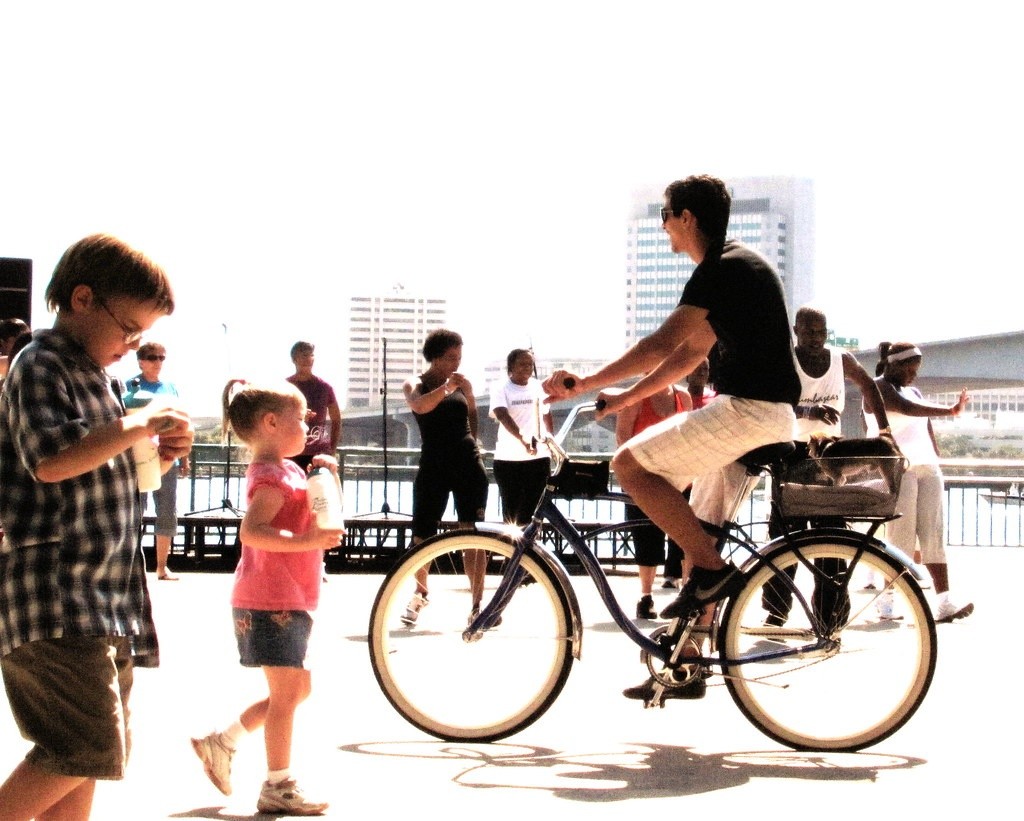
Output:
[780,456,905,517]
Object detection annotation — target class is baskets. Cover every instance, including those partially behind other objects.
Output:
[780,455,908,519]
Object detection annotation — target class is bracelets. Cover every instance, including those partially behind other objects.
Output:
[950,408,957,415]
[803,406,810,419]
[445,378,453,396]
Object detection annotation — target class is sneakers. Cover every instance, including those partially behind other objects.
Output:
[622,673,708,701]
[255,776,329,816]
[658,560,748,619]
[636,595,658,620]
[660,574,678,590]
[190,732,237,797]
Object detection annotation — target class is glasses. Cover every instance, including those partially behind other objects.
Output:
[143,355,166,363]
[89,290,142,345]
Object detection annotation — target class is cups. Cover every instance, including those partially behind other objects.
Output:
[127,408,162,492]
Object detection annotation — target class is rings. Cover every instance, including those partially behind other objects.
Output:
[824,413,829,417]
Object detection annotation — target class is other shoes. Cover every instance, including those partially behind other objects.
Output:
[399,591,430,625]
[467,609,502,629]
[764,614,785,643]
[933,600,975,623]
[874,596,906,621]
[157,566,180,580]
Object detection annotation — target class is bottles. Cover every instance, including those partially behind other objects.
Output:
[307,464,344,535]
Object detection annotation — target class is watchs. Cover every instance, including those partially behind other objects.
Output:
[880,426,892,434]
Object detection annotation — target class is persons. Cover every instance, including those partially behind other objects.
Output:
[402,329,502,628]
[121,342,191,580]
[861,341,974,624]
[286,342,342,582]
[488,348,554,528]
[541,175,803,699]
[191,376,346,815]
[616,357,715,622]
[0,318,33,399]
[0,234,197,821]
[762,308,899,650]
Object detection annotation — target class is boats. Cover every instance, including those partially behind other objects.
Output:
[980,493,1024,506]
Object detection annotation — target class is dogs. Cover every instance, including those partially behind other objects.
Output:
[805,431,899,492]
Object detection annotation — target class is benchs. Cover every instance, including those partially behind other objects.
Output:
[139,514,634,569]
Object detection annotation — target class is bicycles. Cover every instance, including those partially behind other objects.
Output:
[367,378,940,753]
